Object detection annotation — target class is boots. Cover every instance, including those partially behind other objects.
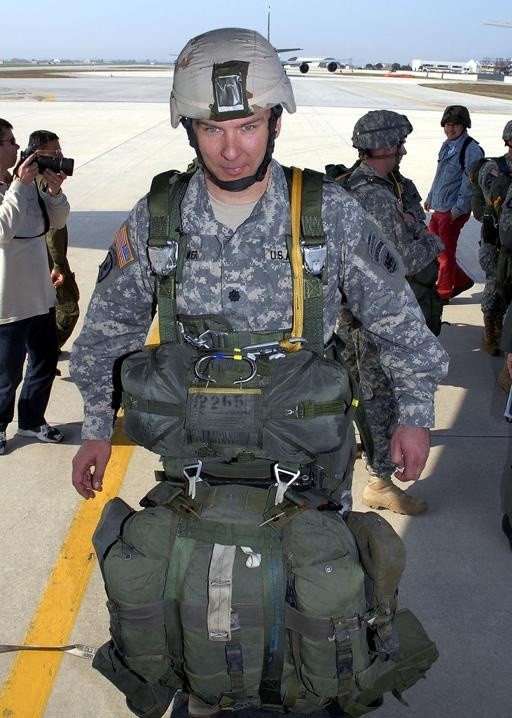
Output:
[482,315,504,357]
[496,352,512,394]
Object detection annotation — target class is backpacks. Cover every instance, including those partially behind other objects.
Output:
[469,157,509,224]
[92,168,439,718]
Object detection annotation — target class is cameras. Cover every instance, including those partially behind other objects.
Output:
[20,145,74,176]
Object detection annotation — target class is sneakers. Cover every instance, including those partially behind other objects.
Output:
[442,298,449,305]
[18,424,65,443]
[451,279,474,298]
[361,477,430,517]
[0,431,8,455]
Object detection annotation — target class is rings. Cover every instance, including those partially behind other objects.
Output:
[53,180,58,184]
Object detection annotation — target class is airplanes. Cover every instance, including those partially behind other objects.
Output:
[165,1,358,75]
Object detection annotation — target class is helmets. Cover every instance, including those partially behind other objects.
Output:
[440,105,471,130]
[170,28,296,129]
[502,120,512,148]
[351,108,413,149]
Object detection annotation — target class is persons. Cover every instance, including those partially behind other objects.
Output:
[477,118,511,359]
[495,301,512,554]
[66,25,449,718]
[1,117,71,455]
[328,108,444,515]
[23,128,82,376]
[422,103,486,302]
